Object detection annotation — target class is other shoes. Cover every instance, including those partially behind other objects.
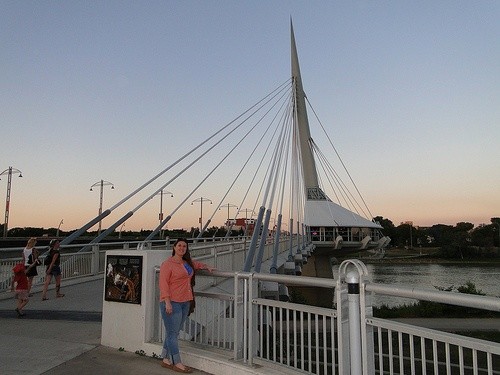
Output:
[161,361,173,369]
[173,365,193,373]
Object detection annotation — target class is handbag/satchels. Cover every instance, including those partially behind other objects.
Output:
[27,248,42,266]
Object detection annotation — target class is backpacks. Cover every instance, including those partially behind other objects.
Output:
[44,255,52,265]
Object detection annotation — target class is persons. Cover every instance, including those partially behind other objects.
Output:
[40,239,65,300]
[159,238,217,373]
[22,238,40,297]
[11,263,29,316]
